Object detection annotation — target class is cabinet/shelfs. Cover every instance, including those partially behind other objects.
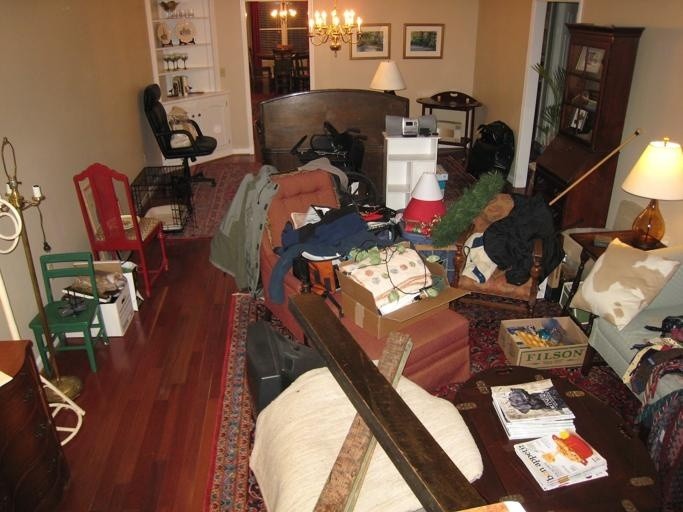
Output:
[144,0,233,174]
[0,339,74,512]
[532,23,646,303]
[381,131,442,210]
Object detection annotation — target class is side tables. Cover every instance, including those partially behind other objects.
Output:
[560,229,668,338]
[416,91,483,159]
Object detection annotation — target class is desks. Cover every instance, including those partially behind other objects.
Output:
[256,50,309,84]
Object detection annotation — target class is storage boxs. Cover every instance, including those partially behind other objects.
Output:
[335,241,471,341]
[398,221,455,284]
[436,164,449,199]
[497,316,589,370]
[64,281,135,337]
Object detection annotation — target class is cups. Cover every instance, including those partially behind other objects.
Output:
[166,6,177,20]
[178,7,186,18]
[187,6,194,18]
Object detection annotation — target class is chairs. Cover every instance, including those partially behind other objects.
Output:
[28,251,110,374]
[73,162,169,299]
[247,48,272,88]
[144,83,217,187]
[273,49,293,90]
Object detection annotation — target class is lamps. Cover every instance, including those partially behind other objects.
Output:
[270,0,296,21]
[308,0,363,59]
[620,137,683,249]
[369,60,406,96]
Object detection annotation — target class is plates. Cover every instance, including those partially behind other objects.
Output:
[119,214,140,230]
[174,22,197,43]
[157,24,173,45]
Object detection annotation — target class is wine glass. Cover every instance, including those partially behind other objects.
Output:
[181,52,189,71]
[169,54,178,71]
[173,51,181,69]
[162,54,171,72]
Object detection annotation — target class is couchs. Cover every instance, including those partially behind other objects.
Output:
[261,169,340,345]
[449,192,543,319]
[581,245,683,447]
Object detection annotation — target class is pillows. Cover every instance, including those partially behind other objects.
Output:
[570,237,682,332]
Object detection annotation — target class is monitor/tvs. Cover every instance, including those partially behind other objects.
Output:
[244,320,321,413]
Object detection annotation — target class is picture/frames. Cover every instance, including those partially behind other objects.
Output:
[403,23,445,59]
[349,23,391,60]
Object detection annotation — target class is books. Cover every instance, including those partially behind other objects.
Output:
[488,377,580,438]
[510,432,608,493]
[572,46,610,72]
[569,103,596,133]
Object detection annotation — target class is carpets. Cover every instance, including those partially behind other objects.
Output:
[204,291,642,512]
[156,154,261,239]
